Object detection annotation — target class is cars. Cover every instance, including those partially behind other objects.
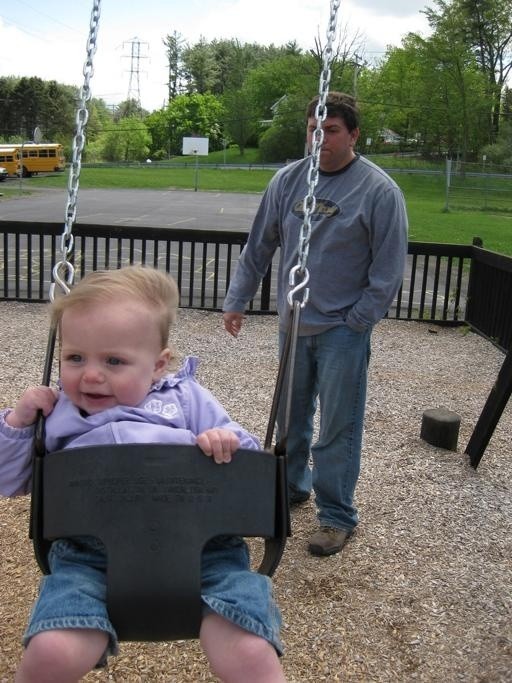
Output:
[375,130,419,146]
[0,166,8,180]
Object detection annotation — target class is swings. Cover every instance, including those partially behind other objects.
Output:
[28,0,338,642]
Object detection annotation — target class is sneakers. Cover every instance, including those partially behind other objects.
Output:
[308,525,349,556]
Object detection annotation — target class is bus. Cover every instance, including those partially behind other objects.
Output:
[0,148,19,177]
[0,142,64,176]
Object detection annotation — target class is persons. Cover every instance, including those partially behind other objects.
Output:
[221,90,408,557]
[1,262,290,682]
